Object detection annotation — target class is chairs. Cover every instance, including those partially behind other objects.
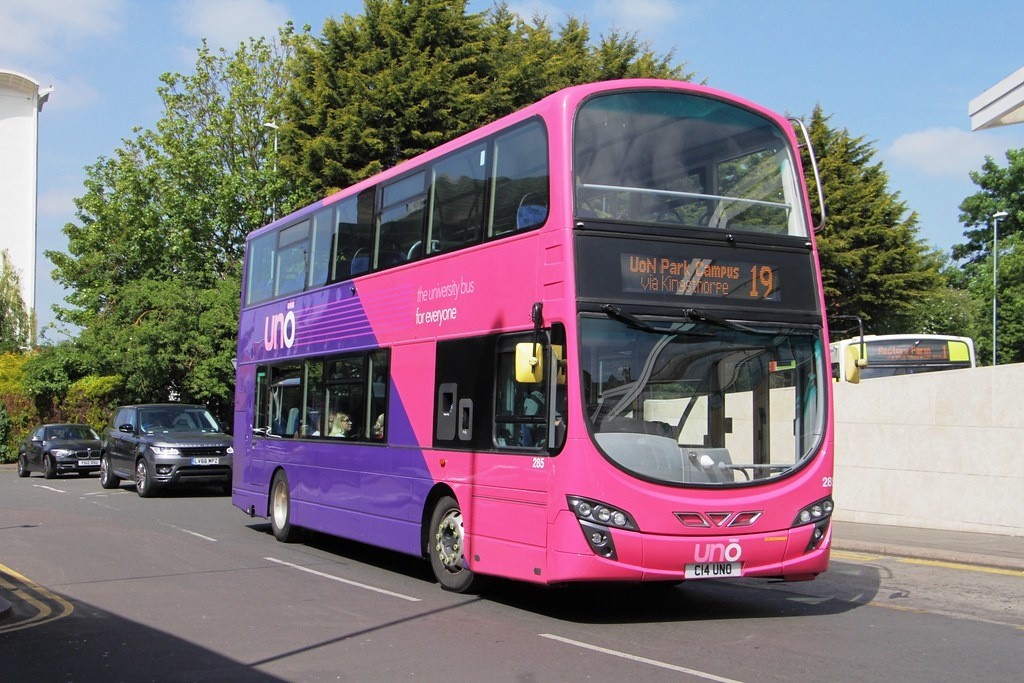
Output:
[521,423,535,447]
[251,239,410,304]
[516,194,549,229]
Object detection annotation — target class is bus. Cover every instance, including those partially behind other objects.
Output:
[828,332,977,384]
[271,377,386,443]
[231,78,869,592]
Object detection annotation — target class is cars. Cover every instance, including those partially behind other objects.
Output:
[18,423,104,479]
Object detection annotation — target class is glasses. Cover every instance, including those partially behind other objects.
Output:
[341,419,351,423]
[329,420,334,423]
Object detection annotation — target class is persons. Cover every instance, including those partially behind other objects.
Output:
[175,419,188,429]
[554,368,599,451]
[311,412,334,436]
[327,411,353,437]
[373,413,384,439]
[144,414,163,430]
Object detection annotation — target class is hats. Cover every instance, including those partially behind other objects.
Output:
[374,414,384,429]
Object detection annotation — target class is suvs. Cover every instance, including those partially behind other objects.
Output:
[96,401,233,495]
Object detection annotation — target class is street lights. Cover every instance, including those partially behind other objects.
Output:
[992,211,1009,366]
[263,122,279,220]
